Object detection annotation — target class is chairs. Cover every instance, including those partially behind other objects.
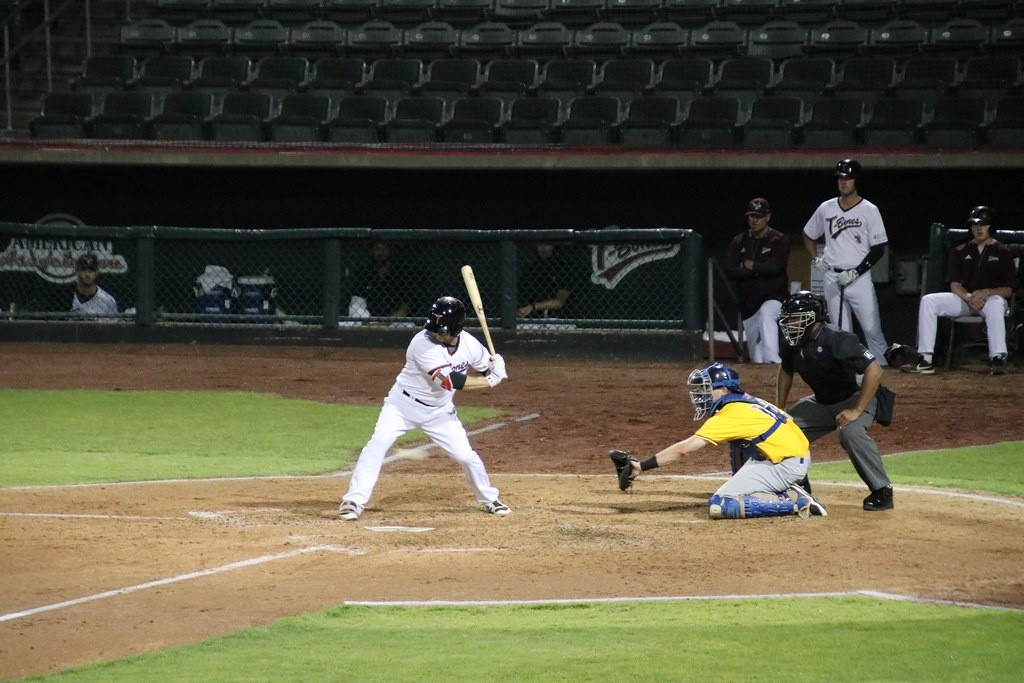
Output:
[26,0,1024,161]
[944,242,1021,369]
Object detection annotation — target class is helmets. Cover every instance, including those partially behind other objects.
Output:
[833,158,862,180]
[966,205,998,239]
[781,291,832,352]
[423,296,466,337]
[692,365,744,395]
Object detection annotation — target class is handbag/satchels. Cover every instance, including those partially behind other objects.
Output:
[876,383,896,427]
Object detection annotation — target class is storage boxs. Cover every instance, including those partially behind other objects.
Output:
[198,286,234,314]
[237,276,288,317]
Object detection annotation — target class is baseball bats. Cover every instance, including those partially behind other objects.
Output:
[838,284,844,330]
[461,264,496,356]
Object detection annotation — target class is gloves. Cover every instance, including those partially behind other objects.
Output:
[812,257,829,273]
[485,353,508,388]
[835,269,859,286]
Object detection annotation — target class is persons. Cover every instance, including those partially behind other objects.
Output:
[338,296,513,521]
[629,362,830,517]
[339,239,412,325]
[515,240,603,319]
[66,254,118,322]
[900,205,1018,375]
[724,197,791,366]
[777,291,893,511]
[800,157,892,366]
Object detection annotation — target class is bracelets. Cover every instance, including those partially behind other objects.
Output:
[532,302,536,312]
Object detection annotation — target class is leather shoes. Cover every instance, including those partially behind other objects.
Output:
[863,485,894,511]
[799,474,811,494]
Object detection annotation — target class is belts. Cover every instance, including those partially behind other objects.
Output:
[402,389,431,406]
[828,267,851,273]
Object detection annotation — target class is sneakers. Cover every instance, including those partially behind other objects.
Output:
[479,498,512,517]
[339,501,359,521]
[786,483,829,516]
[899,355,935,375]
[989,355,1009,376]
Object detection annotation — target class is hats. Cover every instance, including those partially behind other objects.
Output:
[76,254,99,269]
[745,198,770,218]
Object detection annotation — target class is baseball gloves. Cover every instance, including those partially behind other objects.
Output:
[608,448,640,494]
